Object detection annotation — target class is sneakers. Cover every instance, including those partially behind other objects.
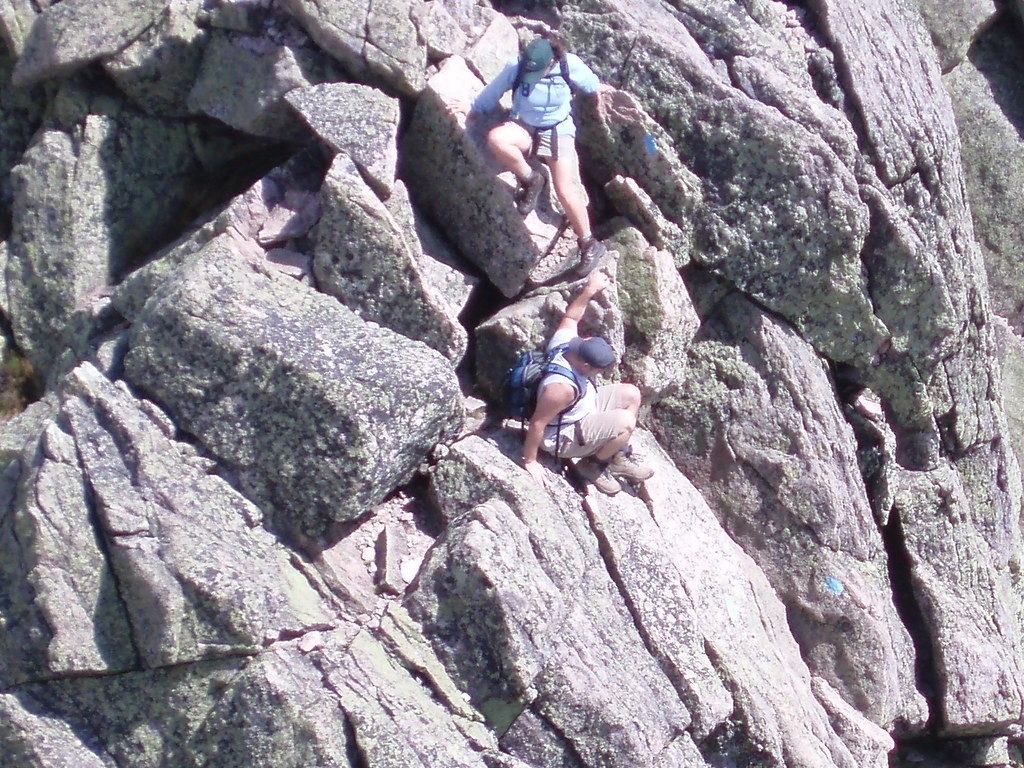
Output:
[575,237,606,278]
[574,457,620,494]
[517,171,547,215]
[610,447,654,480]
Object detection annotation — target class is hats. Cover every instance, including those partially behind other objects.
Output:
[519,39,553,85]
[570,337,615,368]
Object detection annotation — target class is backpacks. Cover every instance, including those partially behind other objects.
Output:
[497,342,588,421]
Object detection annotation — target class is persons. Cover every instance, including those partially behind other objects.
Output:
[522,268,655,494]
[445,38,608,278]
